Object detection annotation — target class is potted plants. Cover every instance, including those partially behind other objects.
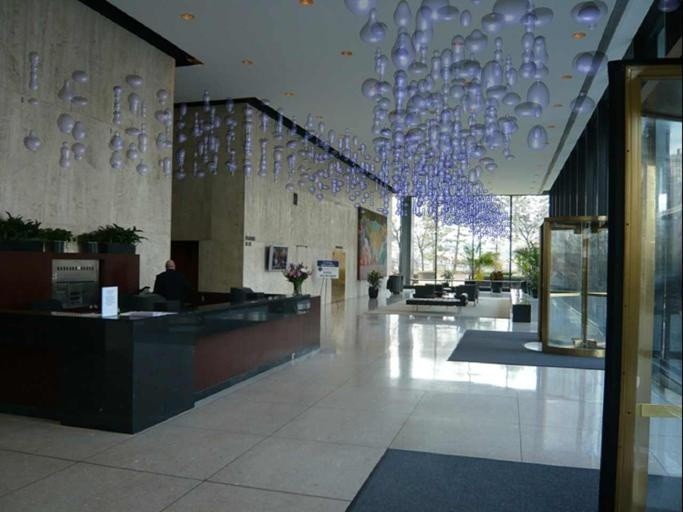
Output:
[76,229,100,252]
[364,270,382,299]
[0,212,44,251]
[514,243,539,298]
[97,225,148,254]
[41,226,73,253]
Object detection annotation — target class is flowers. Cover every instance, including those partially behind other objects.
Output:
[283,261,309,280]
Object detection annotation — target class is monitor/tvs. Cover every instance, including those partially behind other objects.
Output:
[268,244,290,272]
[231,287,264,304]
[129,293,181,314]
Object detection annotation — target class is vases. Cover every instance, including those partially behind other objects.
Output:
[291,281,302,295]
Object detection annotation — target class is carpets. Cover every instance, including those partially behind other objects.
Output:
[444,328,607,370]
[342,446,683,510]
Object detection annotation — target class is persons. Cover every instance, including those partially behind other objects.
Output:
[154,260,183,294]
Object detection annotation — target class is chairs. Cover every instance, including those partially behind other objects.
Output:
[386,274,532,324]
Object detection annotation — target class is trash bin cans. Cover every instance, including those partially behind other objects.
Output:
[387,275,404,294]
